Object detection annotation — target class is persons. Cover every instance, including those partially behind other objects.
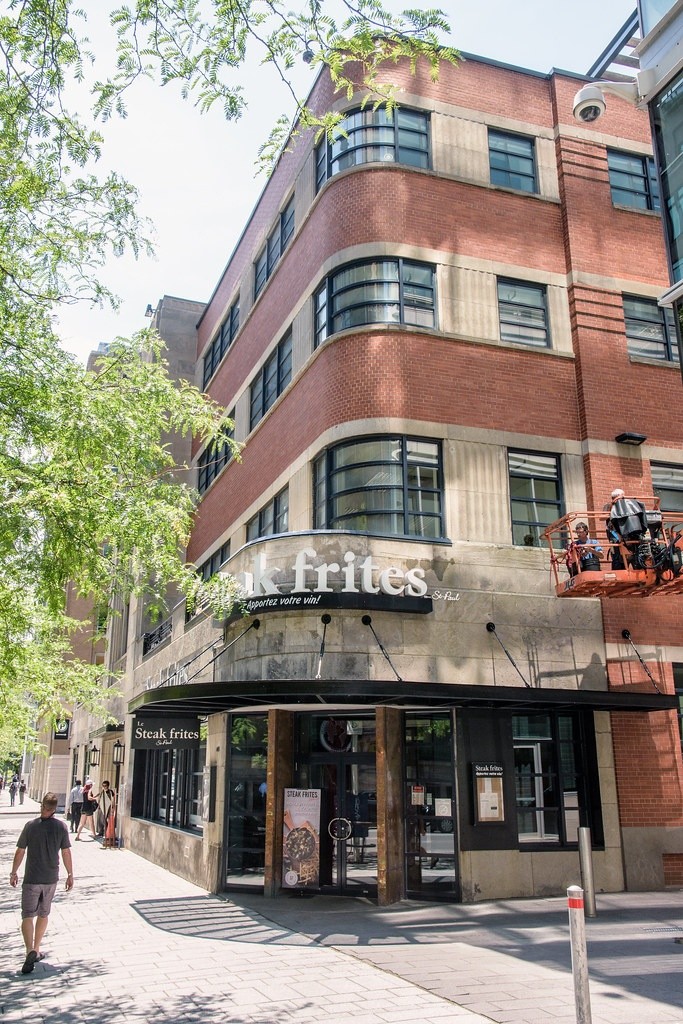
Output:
[10,792,73,974]
[0,774,25,807]
[75,780,100,841]
[68,779,84,833]
[599,489,626,571]
[566,522,604,575]
[92,781,115,837]
[520,535,535,546]
[405,794,439,870]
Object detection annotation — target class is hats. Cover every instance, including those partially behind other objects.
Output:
[86,780,95,785]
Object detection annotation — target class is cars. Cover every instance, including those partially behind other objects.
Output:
[333,789,455,838]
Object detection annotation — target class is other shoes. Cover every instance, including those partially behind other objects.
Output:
[21,951,38,973]
[35,952,45,962]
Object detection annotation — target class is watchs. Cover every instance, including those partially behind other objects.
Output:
[9,873,17,878]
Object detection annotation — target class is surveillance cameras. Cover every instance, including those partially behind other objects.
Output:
[573,87,606,123]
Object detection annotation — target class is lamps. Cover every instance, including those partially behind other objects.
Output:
[613,430,650,445]
[113,738,125,767]
[88,744,100,766]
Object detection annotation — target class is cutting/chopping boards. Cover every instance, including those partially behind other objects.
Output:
[283,809,319,866]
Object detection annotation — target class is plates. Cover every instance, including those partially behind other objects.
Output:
[285,827,316,860]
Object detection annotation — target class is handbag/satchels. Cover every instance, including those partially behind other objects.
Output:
[85,799,98,813]
[67,811,71,821]
